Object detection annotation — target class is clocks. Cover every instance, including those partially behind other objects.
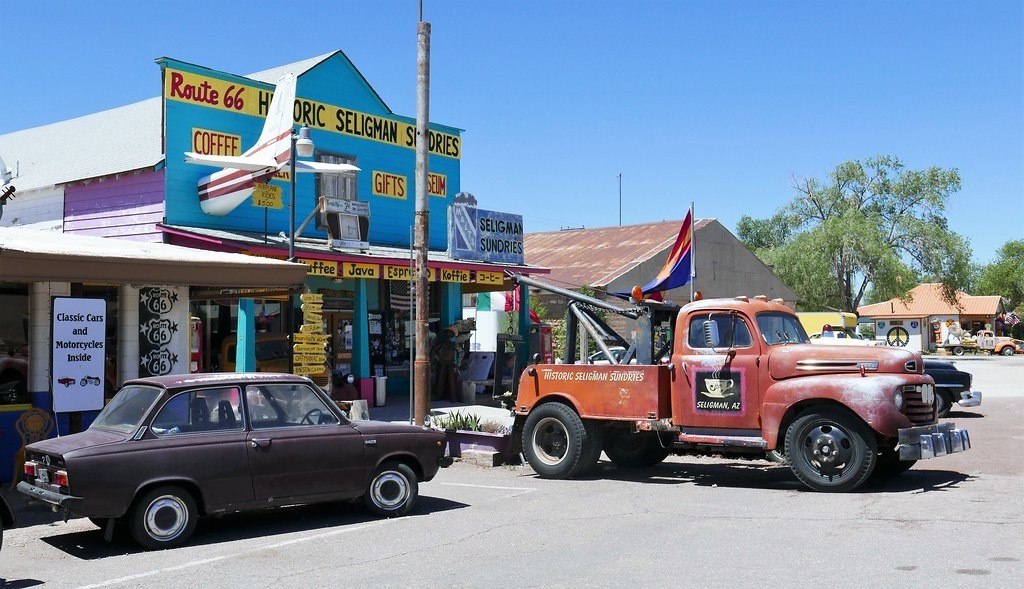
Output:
[339,213,361,241]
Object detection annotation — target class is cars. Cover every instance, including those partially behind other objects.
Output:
[923,359,983,419]
[19,370,448,551]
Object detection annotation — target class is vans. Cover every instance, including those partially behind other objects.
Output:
[772,309,861,342]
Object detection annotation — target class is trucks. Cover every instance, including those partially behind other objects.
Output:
[934,329,1016,356]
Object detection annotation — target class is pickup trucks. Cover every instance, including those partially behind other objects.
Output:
[515,295,941,492]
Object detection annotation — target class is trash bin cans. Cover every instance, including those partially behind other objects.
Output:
[356,377,374,407]
[371,376,388,407]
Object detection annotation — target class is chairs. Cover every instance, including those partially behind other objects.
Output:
[219,400,236,427]
[10,408,54,491]
[192,398,209,425]
